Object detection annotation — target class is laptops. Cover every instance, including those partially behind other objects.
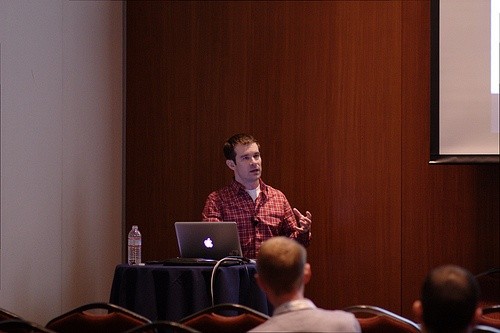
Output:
[174,222,258,261]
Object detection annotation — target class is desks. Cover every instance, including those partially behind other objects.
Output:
[108,265,272,322]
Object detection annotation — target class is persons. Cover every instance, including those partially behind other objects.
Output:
[414,265,475,333]
[246,236,362,333]
[198,132,313,260]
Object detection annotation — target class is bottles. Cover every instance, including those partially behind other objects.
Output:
[128,225,141,266]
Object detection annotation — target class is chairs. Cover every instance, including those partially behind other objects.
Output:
[0,301,500,333]
